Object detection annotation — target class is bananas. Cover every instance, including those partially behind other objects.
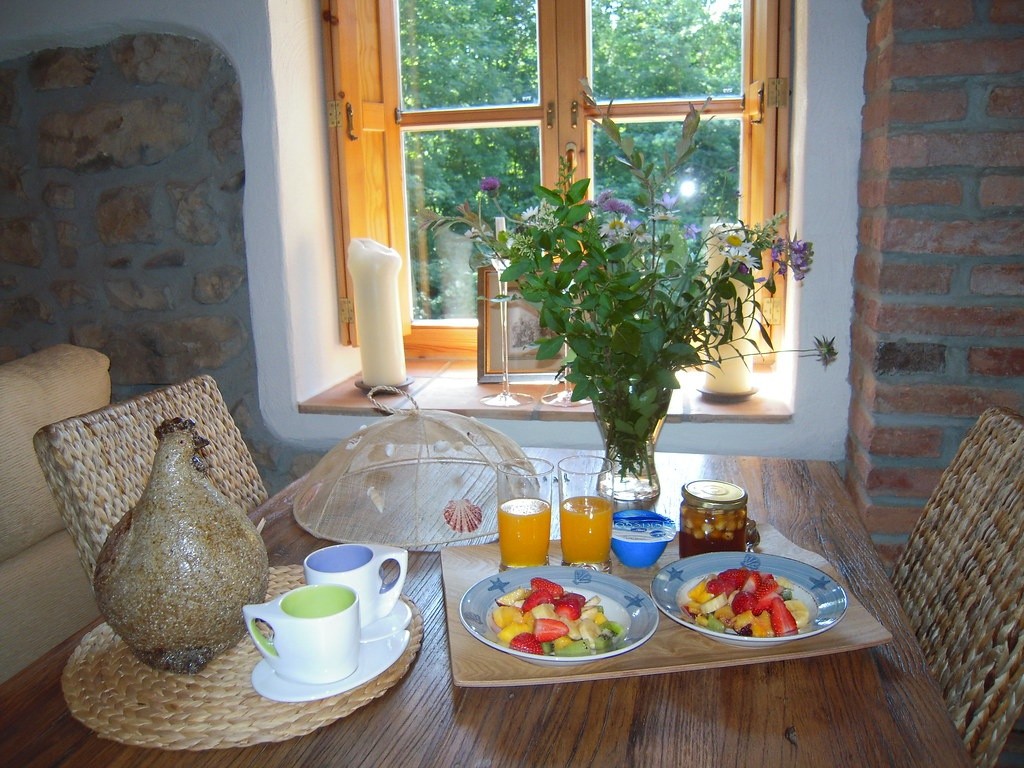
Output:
[784,600,810,627]
[700,591,727,613]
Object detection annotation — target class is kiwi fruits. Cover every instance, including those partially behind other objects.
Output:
[541,621,623,655]
[708,614,726,633]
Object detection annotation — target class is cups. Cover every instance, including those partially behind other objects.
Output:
[302,541,409,627]
[495,457,554,573]
[556,455,615,577]
[241,582,360,685]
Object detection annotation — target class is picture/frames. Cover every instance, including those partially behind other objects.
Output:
[476,264,569,386]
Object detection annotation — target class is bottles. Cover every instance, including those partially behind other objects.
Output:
[678,479,748,558]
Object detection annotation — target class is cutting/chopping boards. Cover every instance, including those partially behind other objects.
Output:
[439,519,894,689]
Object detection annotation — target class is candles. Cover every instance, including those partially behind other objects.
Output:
[698,222,755,393]
[346,238,407,386]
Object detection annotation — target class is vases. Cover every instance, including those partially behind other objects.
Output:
[595,387,671,502]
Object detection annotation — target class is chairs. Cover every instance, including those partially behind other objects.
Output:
[887,406,1024,768]
[31,374,268,612]
[0,340,123,683]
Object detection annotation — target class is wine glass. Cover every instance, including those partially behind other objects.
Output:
[472,239,595,407]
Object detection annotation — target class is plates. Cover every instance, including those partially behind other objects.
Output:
[357,598,412,646]
[250,630,411,705]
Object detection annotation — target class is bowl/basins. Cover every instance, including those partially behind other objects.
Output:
[458,566,660,668]
[612,509,676,568]
[648,550,848,650]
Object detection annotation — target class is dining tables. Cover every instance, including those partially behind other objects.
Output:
[0,443,981,768]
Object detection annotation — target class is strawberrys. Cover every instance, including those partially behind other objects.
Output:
[705,568,797,636]
[508,577,585,654]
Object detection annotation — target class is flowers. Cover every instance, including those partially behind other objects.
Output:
[412,78,838,483]
